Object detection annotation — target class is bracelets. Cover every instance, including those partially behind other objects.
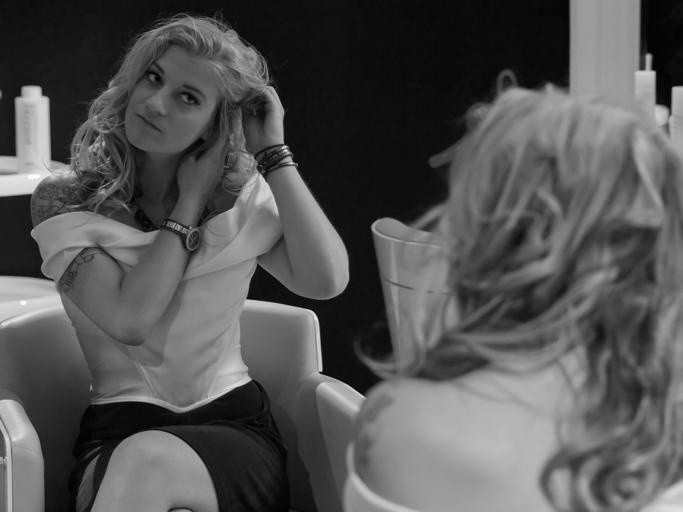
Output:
[253,142,287,161]
[256,146,299,174]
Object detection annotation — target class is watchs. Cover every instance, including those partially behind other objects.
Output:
[161,215,201,253]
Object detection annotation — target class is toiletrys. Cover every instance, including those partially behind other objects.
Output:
[13,85,52,174]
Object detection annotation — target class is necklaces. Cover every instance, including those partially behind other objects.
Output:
[126,186,157,232]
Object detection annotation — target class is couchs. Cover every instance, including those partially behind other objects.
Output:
[368,216,452,373]
[0,297,368,510]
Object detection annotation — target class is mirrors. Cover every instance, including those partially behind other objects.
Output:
[0,0,565,510]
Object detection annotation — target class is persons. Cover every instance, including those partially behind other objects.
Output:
[342,82,681,511]
[24,10,353,511]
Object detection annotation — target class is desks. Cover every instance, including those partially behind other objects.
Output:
[0,154,73,198]
